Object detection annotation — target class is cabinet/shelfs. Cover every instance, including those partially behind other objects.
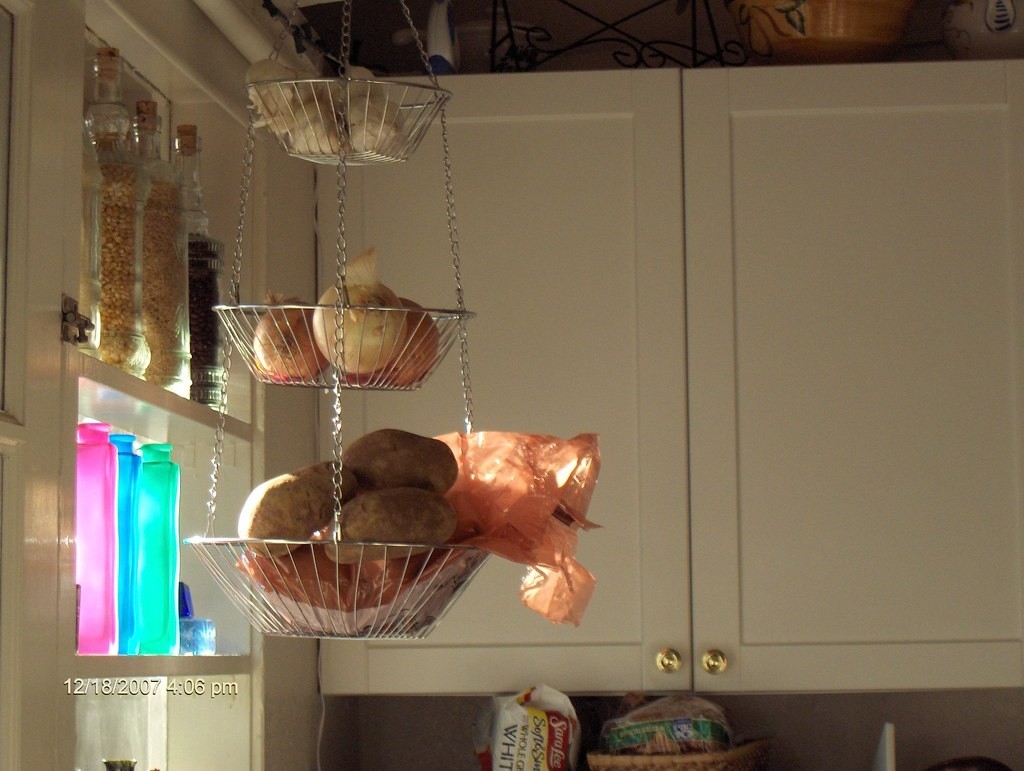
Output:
[75,0,263,771]
[320,55,1024,696]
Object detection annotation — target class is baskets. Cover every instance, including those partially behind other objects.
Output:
[586,736,774,771]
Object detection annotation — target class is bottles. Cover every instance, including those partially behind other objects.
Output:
[135,442,181,656]
[58,63,96,344]
[105,433,140,658]
[129,101,190,390]
[76,421,117,656]
[88,46,154,380]
[169,118,228,415]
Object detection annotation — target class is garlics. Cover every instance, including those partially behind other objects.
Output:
[247,57,408,156]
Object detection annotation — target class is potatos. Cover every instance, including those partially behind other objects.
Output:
[236,428,458,565]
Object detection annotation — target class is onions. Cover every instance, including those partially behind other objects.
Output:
[254,280,440,387]
